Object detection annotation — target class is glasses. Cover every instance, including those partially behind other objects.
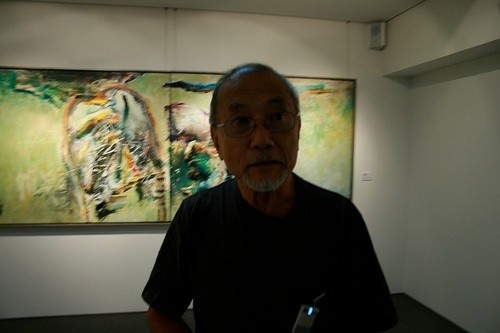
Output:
[216,111,301,138]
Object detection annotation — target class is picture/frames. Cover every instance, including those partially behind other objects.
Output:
[0,66,357,227]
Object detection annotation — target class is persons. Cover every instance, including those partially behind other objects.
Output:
[142,63,398,333]
